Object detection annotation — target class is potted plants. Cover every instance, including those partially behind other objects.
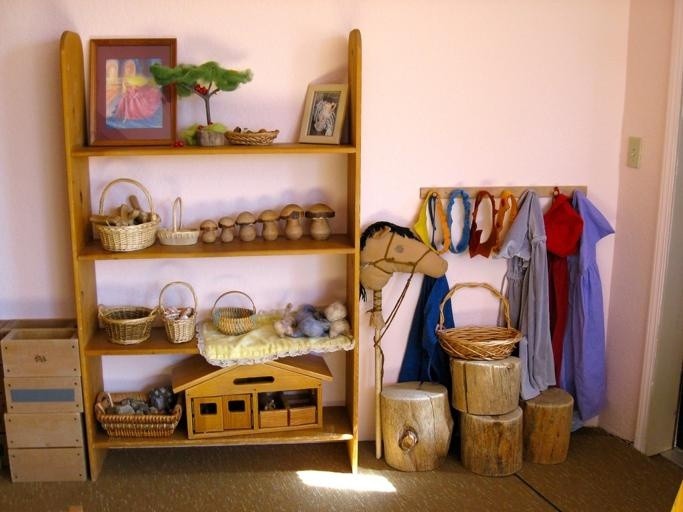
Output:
[148,59,257,147]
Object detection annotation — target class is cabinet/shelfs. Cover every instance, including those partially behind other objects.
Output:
[58,27,364,481]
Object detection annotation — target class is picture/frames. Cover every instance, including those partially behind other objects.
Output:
[298,84,350,146]
[84,37,179,149]
